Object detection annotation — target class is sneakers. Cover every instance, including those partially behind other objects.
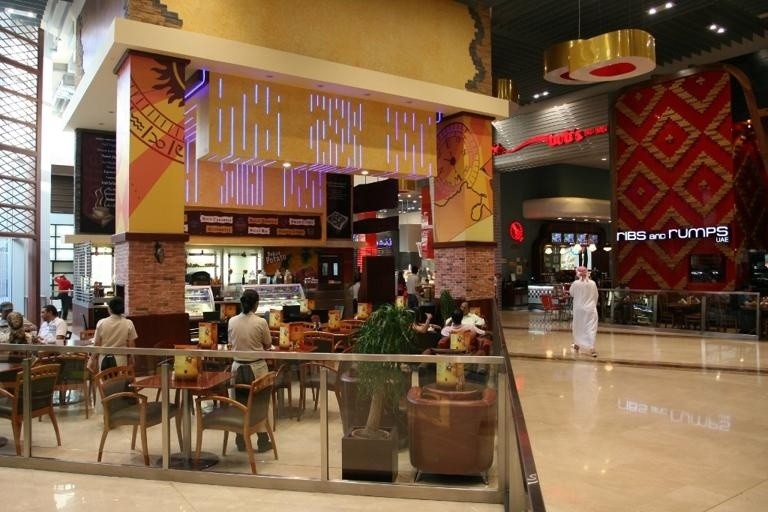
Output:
[258,442,273,453]
[237,444,246,451]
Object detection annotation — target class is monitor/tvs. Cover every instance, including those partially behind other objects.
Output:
[688,253,723,282]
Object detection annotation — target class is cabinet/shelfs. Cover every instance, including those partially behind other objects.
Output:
[235,285,308,320]
[185,285,215,341]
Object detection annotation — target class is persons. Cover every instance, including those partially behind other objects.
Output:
[228,289,273,453]
[569,265,599,357]
[349,266,421,319]
[1,274,138,447]
[502,261,518,311]
[407,302,486,348]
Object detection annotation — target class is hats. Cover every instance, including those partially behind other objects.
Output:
[0,302,12,311]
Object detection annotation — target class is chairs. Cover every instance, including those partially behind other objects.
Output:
[37,350,57,423]
[94,365,180,466]
[292,355,344,420]
[268,364,289,433]
[341,302,420,482]
[539,292,738,334]
[56,348,90,419]
[0,320,492,385]
[196,371,279,475]
[1,363,63,455]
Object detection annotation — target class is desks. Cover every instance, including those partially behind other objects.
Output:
[740,306,768,340]
